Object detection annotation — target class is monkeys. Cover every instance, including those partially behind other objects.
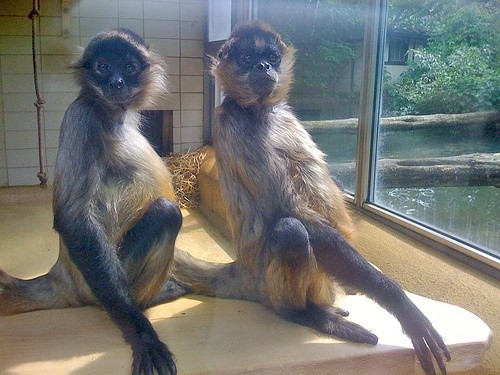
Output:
[169,18,452,375]
[0,27,190,375]
